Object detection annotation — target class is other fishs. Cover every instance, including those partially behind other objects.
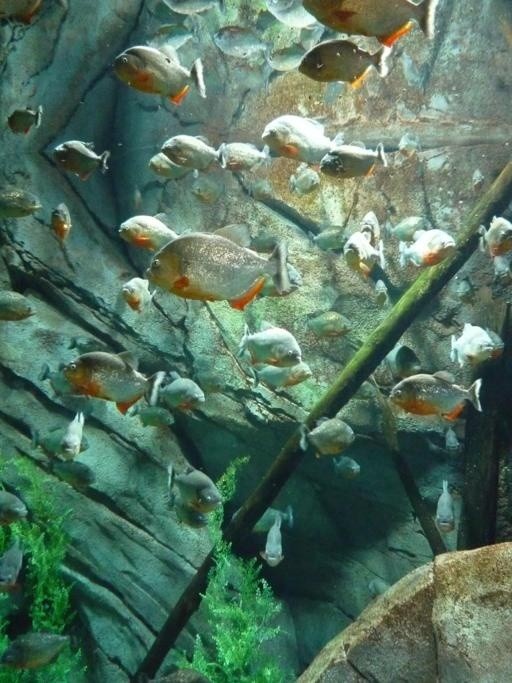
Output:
[0,0,512,460]
